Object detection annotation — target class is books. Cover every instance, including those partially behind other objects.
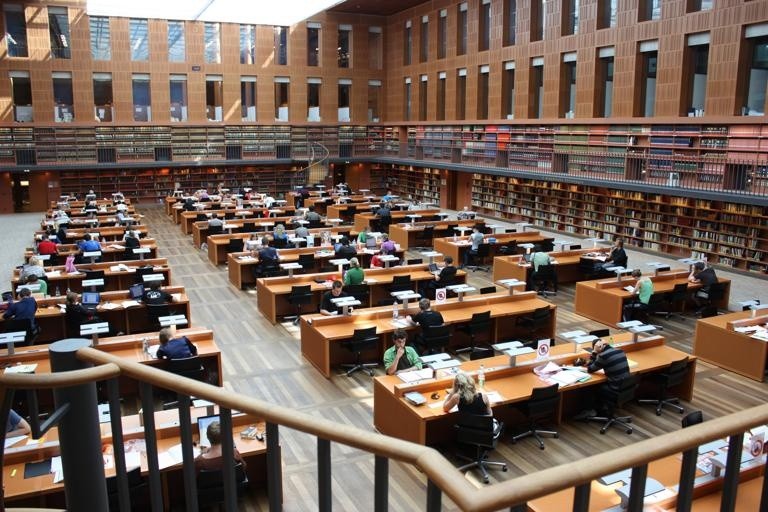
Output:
[627,126,650,154]
[568,153,624,174]
[408,127,459,158]
[650,126,728,183]
[728,125,768,187]
[471,173,508,217]
[171,127,225,157]
[750,206,766,272]
[96,127,172,153]
[1,127,97,161]
[554,127,626,153]
[692,199,749,267]
[565,185,625,241]
[508,177,562,229]
[626,191,665,252]
[460,126,554,168]
[224,128,291,153]
[669,197,692,248]
[291,127,339,155]
[338,128,399,152]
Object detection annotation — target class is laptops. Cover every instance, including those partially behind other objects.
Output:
[429,263,441,275]
[524,253,533,264]
[23,264,30,271]
[365,237,379,250]
[129,283,145,301]
[81,292,100,310]
[372,207,380,214]
[334,243,343,252]
[197,414,236,448]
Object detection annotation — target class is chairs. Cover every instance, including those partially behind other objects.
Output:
[0,184,767,511]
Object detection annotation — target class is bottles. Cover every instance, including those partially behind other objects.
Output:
[53,285,62,297]
[392,300,401,322]
[142,337,148,355]
[478,365,487,388]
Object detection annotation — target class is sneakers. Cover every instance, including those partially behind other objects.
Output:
[574,410,596,419]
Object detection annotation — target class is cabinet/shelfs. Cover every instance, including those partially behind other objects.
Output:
[0,122,384,203]
[383,125,768,280]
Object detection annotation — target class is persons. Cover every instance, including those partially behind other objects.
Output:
[429,257,458,291]
[211,210,400,287]
[64,289,126,337]
[141,281,177,328]
[443,373,500,459]
[383,328,428,375]
[1,400,32,437]
[573,338,629,421]
[158,327,206,377]
[621,269,655,323]
[319,280,357,315]
[527,244,559,296]
[193,420,247,488]
[688,262,721,314]
[1,288,40,348]
[406,298,449,339]
[19,208,145,293]
